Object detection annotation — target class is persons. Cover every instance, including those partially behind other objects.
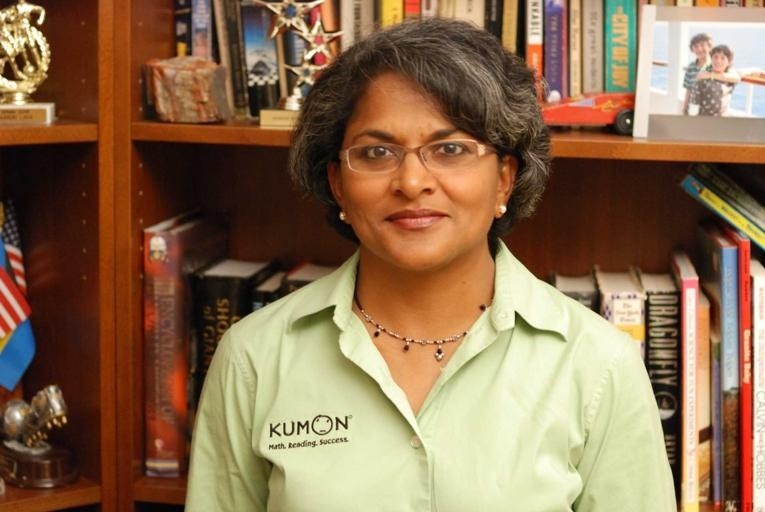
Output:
[184,15,675,512]
[682,33,741,115]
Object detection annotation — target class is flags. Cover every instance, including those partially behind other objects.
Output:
[0,199,36,391]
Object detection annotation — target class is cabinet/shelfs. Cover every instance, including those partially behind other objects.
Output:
[0,0,115,511]
[113,2,765,511]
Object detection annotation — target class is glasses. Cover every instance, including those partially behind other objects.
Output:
[337,139,496,174]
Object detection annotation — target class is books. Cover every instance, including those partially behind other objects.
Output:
[175,0,520,129]
[528,0,765,96]
[143,213,340,477]
[550,163,765,512]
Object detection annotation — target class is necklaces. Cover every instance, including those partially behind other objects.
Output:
[356,292,487,360]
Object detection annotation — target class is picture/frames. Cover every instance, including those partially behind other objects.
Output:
[631,5,765,145]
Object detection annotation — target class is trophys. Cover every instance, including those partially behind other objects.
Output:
[0,0,56,125]
[0,384,80,488]
